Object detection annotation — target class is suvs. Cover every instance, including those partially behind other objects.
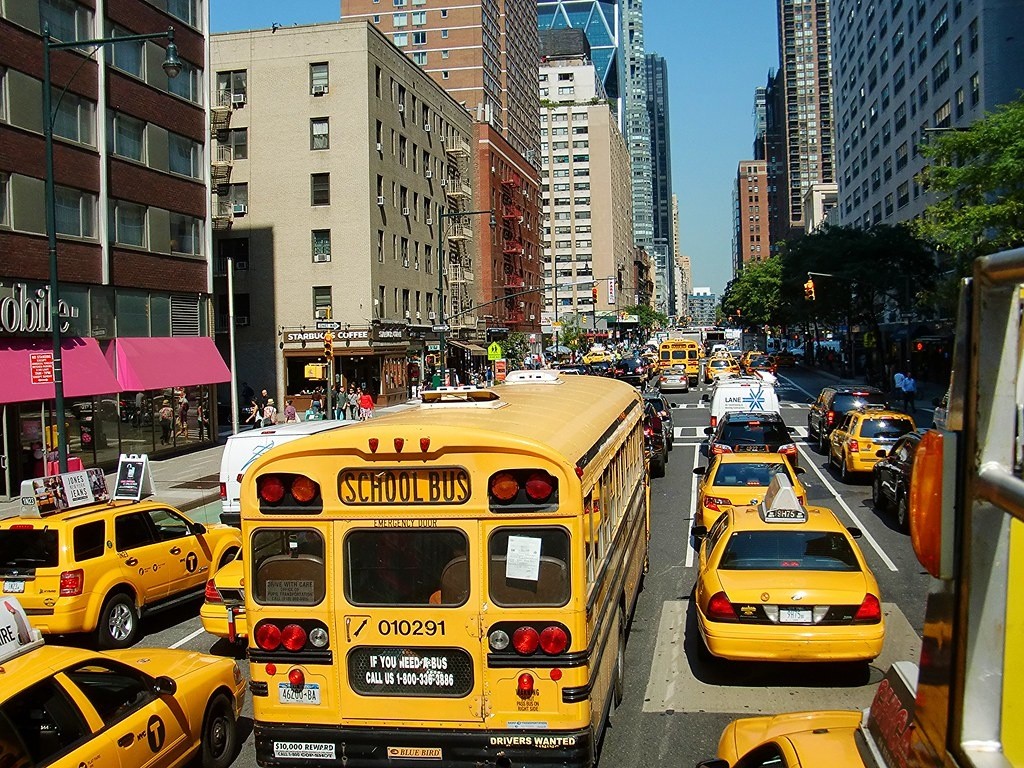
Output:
[870,428,931,532]
[544,327,893,477]
[826,404,917,485]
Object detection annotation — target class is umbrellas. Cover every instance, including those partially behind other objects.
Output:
[546,346,570,353]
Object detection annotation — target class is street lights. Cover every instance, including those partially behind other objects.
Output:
[555,257,589,362]
[437,204,498,390]
[40,20,185,474]
[924,127,981,258]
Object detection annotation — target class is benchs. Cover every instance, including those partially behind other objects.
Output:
[438,553,567,606]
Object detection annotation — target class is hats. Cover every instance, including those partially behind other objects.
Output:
[162,400,170,406]
[267,399,274,405]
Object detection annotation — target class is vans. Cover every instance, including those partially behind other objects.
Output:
[218,419,362,526]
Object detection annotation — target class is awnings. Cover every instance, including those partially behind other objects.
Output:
[450,341,487,355]
[0,337,123,404]
[116,338,231,391]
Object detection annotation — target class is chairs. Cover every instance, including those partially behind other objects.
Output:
[255,554,326,603]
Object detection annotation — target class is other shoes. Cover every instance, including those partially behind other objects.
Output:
[161,440,164,445]
[186,440,192,444]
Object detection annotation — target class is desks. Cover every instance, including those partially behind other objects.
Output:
[34,457,84,478]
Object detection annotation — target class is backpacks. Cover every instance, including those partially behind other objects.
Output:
[258,404,264,417]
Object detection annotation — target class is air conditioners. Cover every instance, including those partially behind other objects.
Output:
[317,254,326,262]
[237,262,247,269]
[232,94,246,104]
[316,309,326,318]
[235,317,247,325]
[232,204,246,214]
[313,85,324,93]
[374,105,457,320]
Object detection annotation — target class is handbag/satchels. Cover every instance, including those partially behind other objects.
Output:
[180,422,188,428]
[295,412,301,423]
[313,401,319,406]
[264,417,271,425]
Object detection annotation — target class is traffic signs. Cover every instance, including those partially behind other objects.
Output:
[316,321,342,332]
[432,324,451,332]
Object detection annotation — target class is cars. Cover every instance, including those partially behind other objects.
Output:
[691,443,810,553]
[0,595,248,768]
[694,661,918,768]
[199,542,247,644]
[1,469,243,650]
[690,472,885,670]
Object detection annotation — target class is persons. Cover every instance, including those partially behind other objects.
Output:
[854,353,867,377]
[894,370,905,401]
[310,382,375,421]
[90,470,101,493]
[136,392,210,445]
[50,477,67,508]
[815,345,842,372]
[550,347,583,363]
[465,366,490,388]
[916,363,928,387]
[902,372,917,413]
[240,382,296,429]
[524,354,541,370]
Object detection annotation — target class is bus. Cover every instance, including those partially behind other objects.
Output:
[907,248,1023,768]
[238,369,667,767]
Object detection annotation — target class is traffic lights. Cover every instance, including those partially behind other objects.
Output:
[803,282,810,300]
[592,287,598,303]
[915,340,927,353]
[582,316,587,324]
[807,280,816,301]
[625,312,629,321]
[426,353,436,366]
[323,332,333,359]
[621,312,625,320]
